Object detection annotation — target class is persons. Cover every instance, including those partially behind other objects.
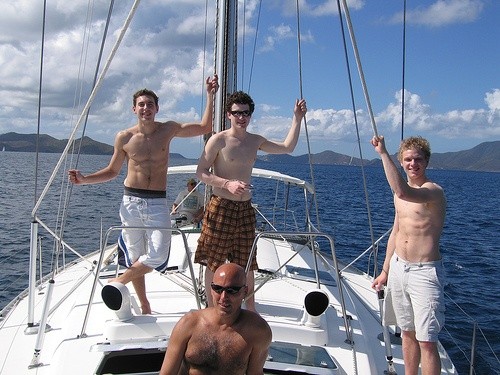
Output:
[371,134,445,375]
[195,90,307,316]
[159,263,272,375]
[68,74,220,314]
[172,178,204,228]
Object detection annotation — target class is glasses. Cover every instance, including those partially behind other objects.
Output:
[230,110,250,117]
[211,282,246,295]
[190,183,196,186]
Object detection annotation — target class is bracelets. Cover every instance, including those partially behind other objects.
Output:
[383,268,388,276]
[223,180,229,187]
